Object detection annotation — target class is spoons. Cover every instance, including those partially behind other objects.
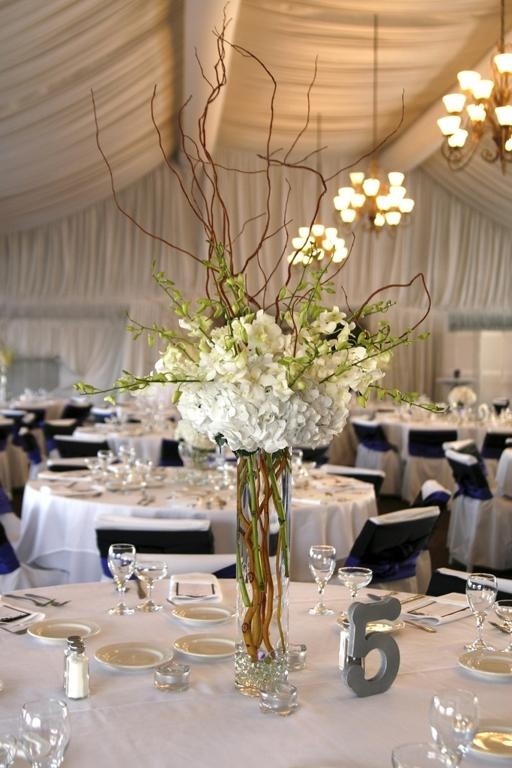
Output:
[367,590,398,601]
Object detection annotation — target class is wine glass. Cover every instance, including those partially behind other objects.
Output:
[390,741,464,768]
[18,696,73,768]
[174,465,237,507]
[463,573,499,653]
[0,732,20,767]
[85,445,153,499]
[137,560,168,613]
[106,543,137,616]
[427,688,481,743]
[307,544,337,617]
[495,599,512,653]
[338,567,374,620]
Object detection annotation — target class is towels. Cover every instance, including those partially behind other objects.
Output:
[402,593,476,628]
[1,601,48,634]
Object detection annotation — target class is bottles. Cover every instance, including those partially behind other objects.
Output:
[66,642,91,700]
[64,635,83,692]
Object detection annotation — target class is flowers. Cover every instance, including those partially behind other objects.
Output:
[71,2,454,669]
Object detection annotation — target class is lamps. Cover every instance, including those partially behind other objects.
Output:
[332,10,416,238]
[286,109,348,273]
[436,0,512,189]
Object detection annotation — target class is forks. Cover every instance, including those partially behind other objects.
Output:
[24,593,72,608]
[4,593,56,609]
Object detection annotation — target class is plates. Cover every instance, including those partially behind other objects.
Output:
[25,617,101,645]
[170,603,236,626]
[456,649,512,684]
[41,481,105,497]
[174,633,233,663]
[93,639,174,674]
[464,724,512,758]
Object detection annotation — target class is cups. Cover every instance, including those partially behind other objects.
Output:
[411,408,512,428]
